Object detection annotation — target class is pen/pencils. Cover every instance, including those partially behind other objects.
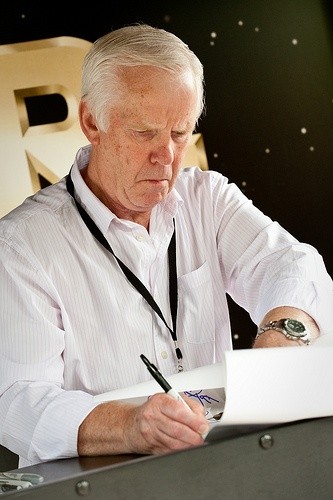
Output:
[140,353,211,439]
[1,480,33,490]
[2,472,44,482]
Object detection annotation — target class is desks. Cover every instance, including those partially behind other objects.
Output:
[0,416,333,500]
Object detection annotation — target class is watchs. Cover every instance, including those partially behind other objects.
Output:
[254,318,310,347]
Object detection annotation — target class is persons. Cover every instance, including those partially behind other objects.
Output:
[0,25,332,462]
